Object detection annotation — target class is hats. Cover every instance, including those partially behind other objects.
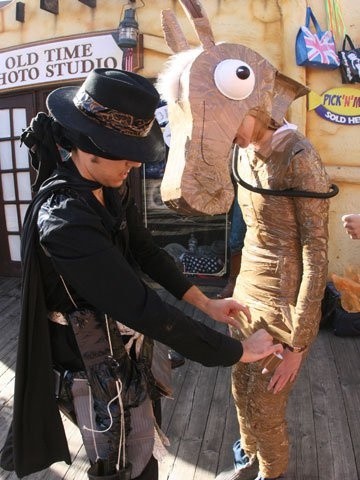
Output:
[46,68,167,161]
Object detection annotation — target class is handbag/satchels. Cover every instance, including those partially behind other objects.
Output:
[338,34,360,85]
[294,6,340,70]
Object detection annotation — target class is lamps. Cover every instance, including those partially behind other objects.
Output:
[116,0,145,49]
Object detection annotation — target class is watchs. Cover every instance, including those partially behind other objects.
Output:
[284,345,307,353]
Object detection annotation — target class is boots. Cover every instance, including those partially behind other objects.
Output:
[214,440,258,480]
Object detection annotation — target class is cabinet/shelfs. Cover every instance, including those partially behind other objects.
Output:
[131,165,231,294]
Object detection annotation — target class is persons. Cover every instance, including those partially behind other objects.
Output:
[215,108,330,480]
[0,66,283,480]
[342,214,360,239]
